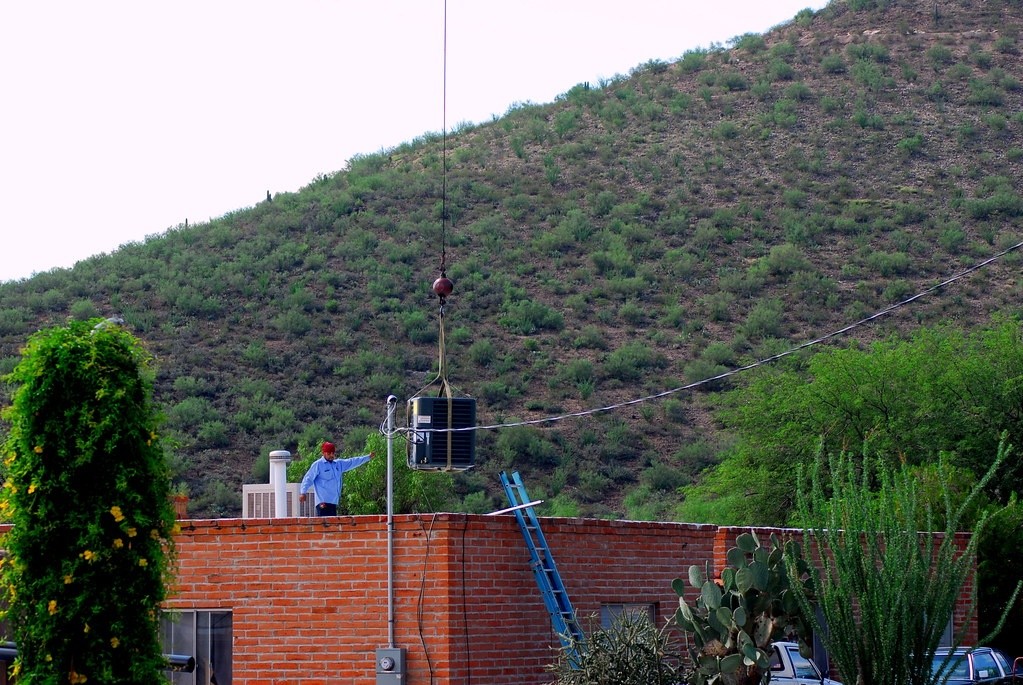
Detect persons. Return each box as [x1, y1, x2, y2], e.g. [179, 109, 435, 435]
[299, 442, 376, 517]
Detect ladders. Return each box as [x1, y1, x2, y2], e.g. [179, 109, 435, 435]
[498, 470, 588, 668]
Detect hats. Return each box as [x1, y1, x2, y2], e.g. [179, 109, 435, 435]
[322, 442, 336, 453]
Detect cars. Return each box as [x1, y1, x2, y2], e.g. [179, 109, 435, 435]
[762, 642, 843, 685]
[908, 645, 1023, 685]
[0, 640, 26, 685]
